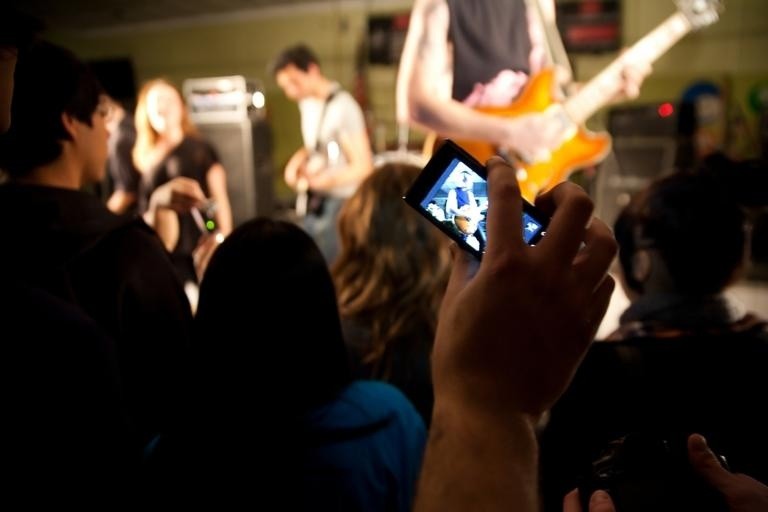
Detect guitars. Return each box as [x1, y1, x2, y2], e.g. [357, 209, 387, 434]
[293, 142, 345, 218]
[423, 0, 725, 220]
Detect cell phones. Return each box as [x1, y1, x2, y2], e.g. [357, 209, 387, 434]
[403, 138, 555, 266]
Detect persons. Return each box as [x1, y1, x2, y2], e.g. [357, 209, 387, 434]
[391, 0, 657, 206]
[444, 172, 487, 254]
[107, 79, 233, 316]
[271, 45, 375, 273]
[1, 35, 768, 511]
[425, 201, 444, 223]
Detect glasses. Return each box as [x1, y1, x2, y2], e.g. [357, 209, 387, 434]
[97, 103, 117, 124]
